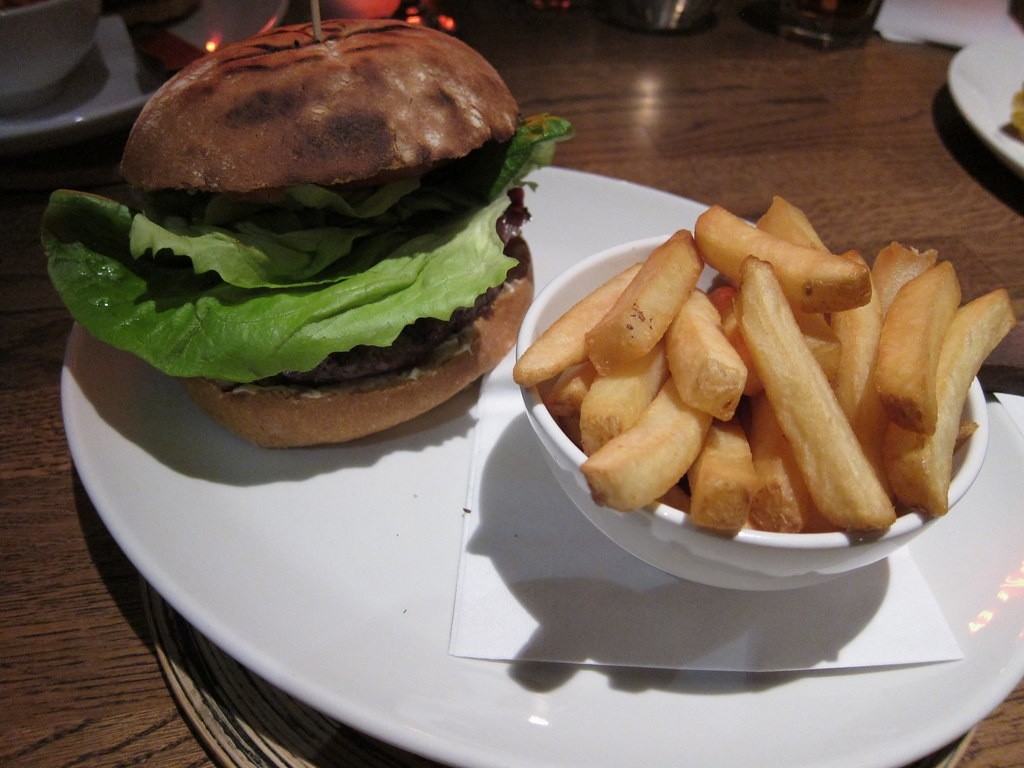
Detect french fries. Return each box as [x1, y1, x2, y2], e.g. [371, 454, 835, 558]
[515, 196, 1013, 535]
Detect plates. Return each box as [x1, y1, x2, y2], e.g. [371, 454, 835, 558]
[0, 0, 293, 147]
[947, 29, 1024, 173]
[61, 161, 1023, 768]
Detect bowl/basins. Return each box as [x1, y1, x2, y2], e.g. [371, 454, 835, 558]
[0, 0, 103, 110]
[517, 233, 992, 592]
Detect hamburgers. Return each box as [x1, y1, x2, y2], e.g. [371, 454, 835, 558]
[43, 25, 570, 449]
